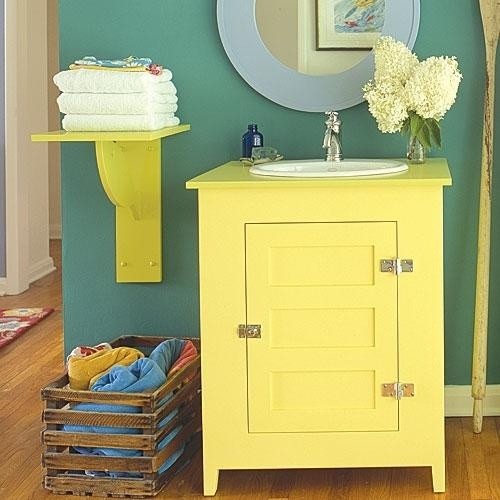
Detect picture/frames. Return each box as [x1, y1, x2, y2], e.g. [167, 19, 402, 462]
[316, 1, 384, 52]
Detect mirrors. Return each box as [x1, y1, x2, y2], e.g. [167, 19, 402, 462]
[216, 0, 422, 114]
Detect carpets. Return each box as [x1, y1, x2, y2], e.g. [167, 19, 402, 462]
[1, 307, 55, 347]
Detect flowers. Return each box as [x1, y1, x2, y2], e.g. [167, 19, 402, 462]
[361, 35, 464, 158]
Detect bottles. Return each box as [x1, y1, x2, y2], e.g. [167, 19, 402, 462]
[241, 124, 263, 160]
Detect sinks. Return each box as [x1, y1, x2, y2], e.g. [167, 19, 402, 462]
[249, 156, 409, 178]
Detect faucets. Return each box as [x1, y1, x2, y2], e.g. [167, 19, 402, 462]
[321, 111, 344, 162]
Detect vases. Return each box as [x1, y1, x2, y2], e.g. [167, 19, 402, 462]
[407, 125, 426, 164]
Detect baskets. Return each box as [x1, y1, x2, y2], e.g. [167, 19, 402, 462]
[39, 336, 202, 498]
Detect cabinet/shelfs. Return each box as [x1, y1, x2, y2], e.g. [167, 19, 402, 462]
[196, 190, 445, 496]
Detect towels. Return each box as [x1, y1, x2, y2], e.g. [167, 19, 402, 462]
[54, 55, 180, 131]
